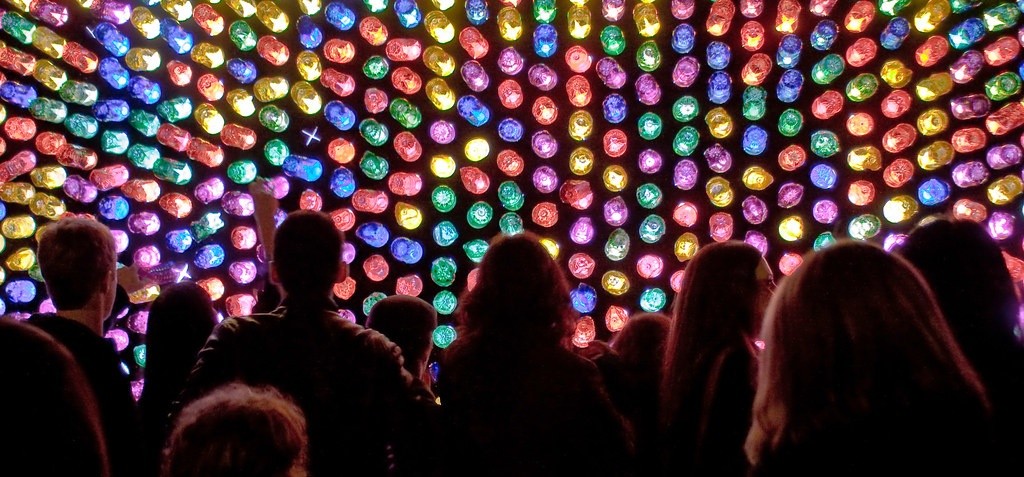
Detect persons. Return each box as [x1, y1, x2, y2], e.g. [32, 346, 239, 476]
[742, 241, 1012, 477]
[20, 217, 137, 477]
[438, 233, 637, 477]
[896, 218, 1024, 444]
[160, 382, 310, 477]
[590, 312, 670, 477]
[136, 282, 219, 477]
[166, 210, 438, 477]
[659, 241, 776, 477]
[0, 314, 111, 477]
[365, 294, 438, 394]
